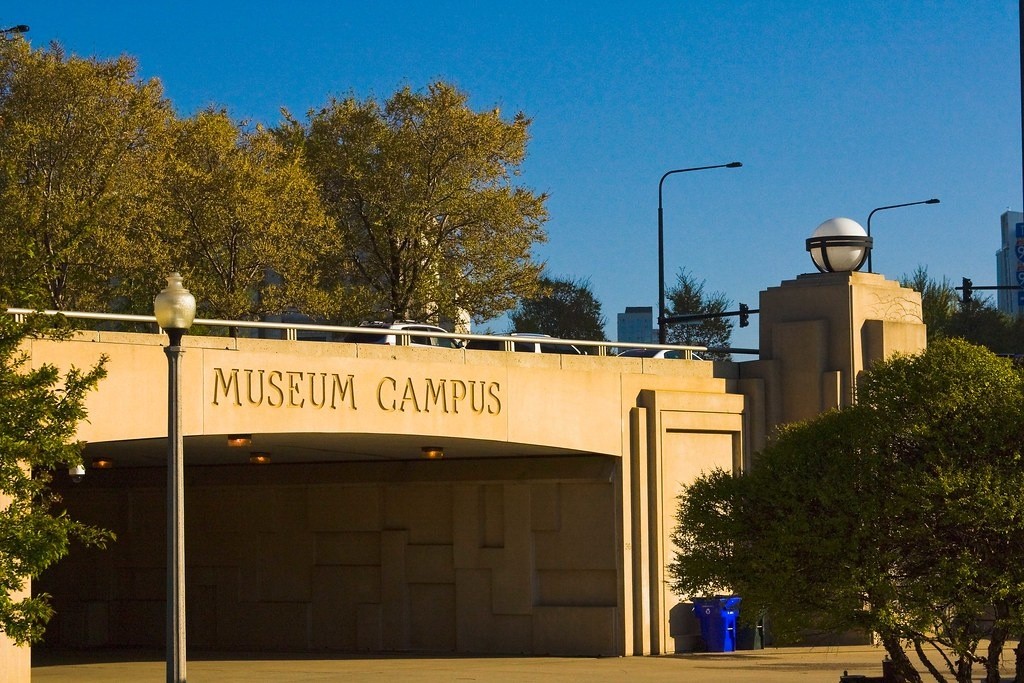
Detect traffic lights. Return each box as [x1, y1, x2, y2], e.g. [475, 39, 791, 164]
[739, 302, 749, 328]
[963, 277, 973, 304]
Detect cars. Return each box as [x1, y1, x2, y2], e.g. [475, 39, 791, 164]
[616, 349, 704, 362]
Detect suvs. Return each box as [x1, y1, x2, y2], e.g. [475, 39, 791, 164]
[466, 333, 587, 356]
[343, 319, 466, 349]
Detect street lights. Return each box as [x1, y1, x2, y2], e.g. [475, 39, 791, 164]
[155, 272, 197, 683]
[657, 162, 743, 345]
[868, 199, 940, 274]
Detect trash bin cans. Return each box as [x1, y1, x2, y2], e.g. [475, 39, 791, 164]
[689, 595, 742, 652]
